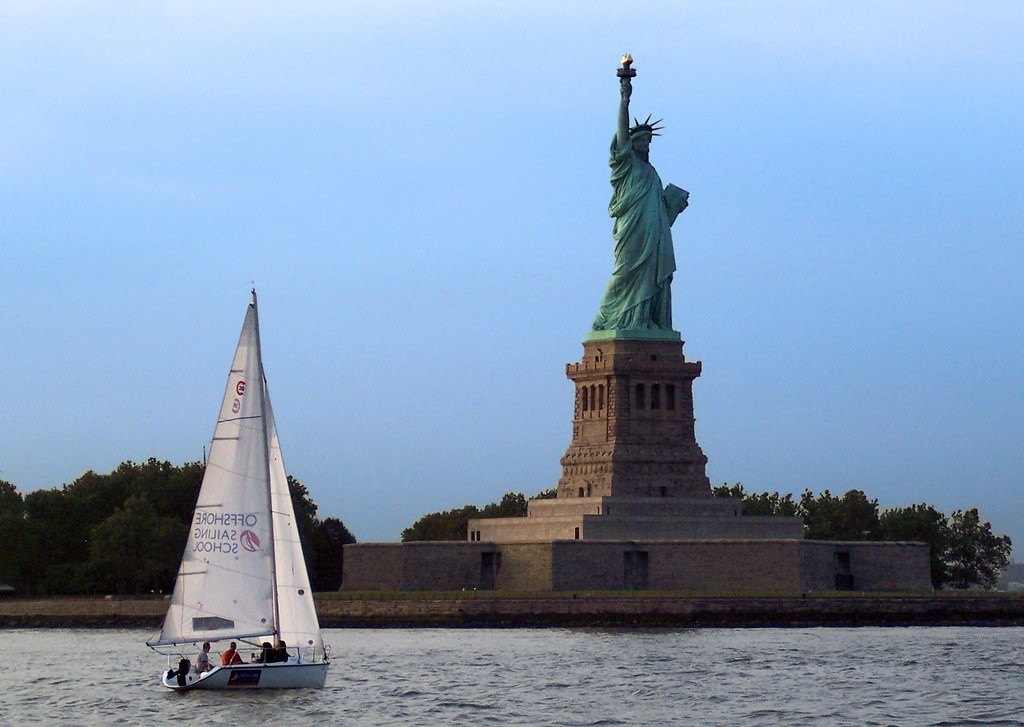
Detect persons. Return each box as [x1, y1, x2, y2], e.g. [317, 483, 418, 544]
[194, 642, 215, 673]
[592, 79, 688, 329]
[256, 642, 282, 663]
[275, 640, 291, 663]
[221, 641, 242, 665]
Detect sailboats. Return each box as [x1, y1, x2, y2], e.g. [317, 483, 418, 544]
[145, 288, 331, 692]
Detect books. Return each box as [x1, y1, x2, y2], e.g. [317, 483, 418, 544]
[663, 183, 690, 227]
